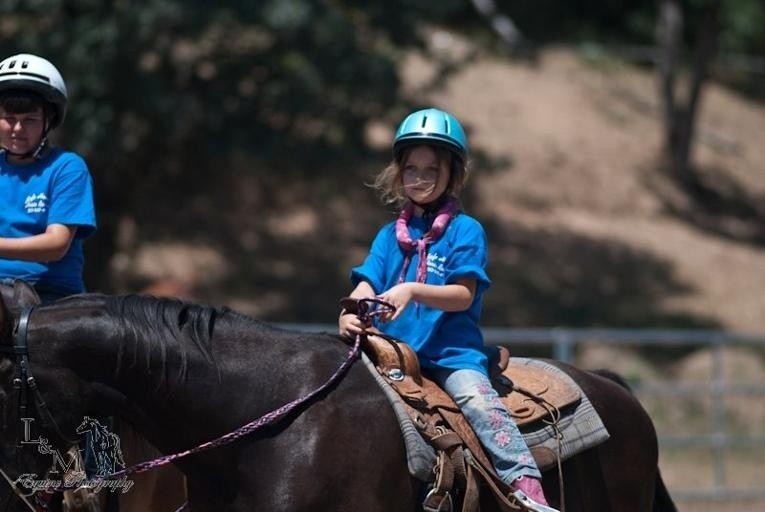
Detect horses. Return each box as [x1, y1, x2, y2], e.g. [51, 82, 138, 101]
[0, 278, 679, 512]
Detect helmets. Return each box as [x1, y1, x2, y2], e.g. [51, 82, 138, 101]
[392, 108, 468, 165]
[0, 53, 68, 129]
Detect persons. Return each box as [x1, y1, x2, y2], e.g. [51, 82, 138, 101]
[336, 105, 553, 510]
[0, 52, 100, 310]
